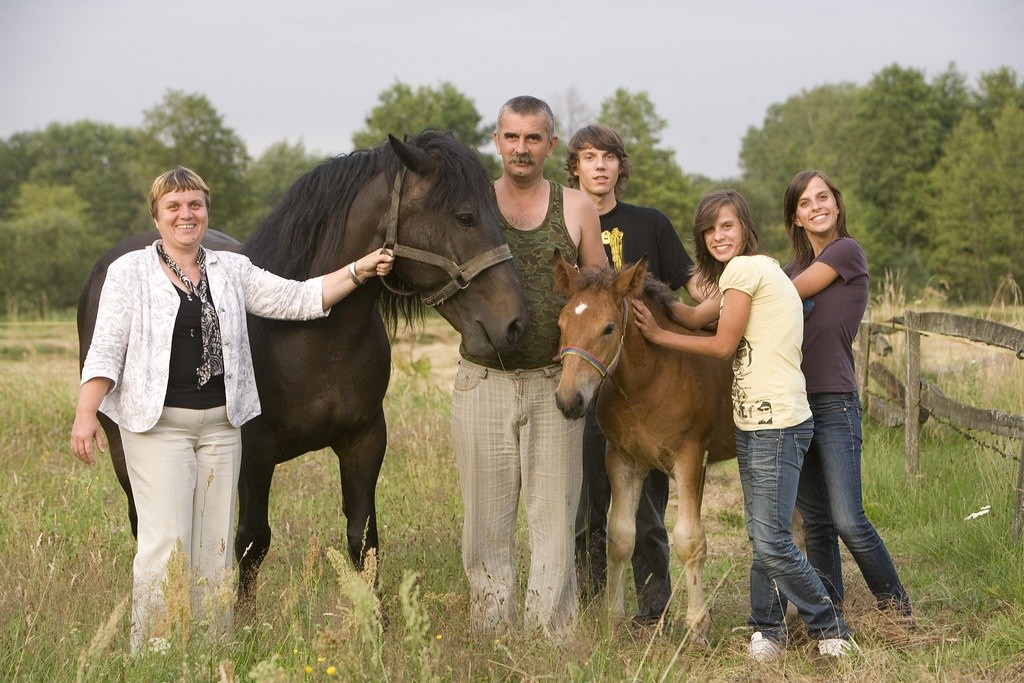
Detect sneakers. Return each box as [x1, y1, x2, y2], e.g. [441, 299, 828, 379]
[747, 631, 781, 665]
[817, 636, 851, 657]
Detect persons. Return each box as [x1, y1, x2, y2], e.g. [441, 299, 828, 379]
[70, 169, 394, 655]
[451, 94, 609, 640]
[563, 125, 716, 629]
[780, 172, 911, 618]
[631, 190, 849, 662]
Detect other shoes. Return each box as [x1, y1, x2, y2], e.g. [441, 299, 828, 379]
[630, 606, 669, 631]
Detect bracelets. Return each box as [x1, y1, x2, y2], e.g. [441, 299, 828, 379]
[349, 262, 367, 286]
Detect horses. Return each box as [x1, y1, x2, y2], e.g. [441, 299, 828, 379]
[551, 246, 740, 650]
[76, 125, 529, 635]
